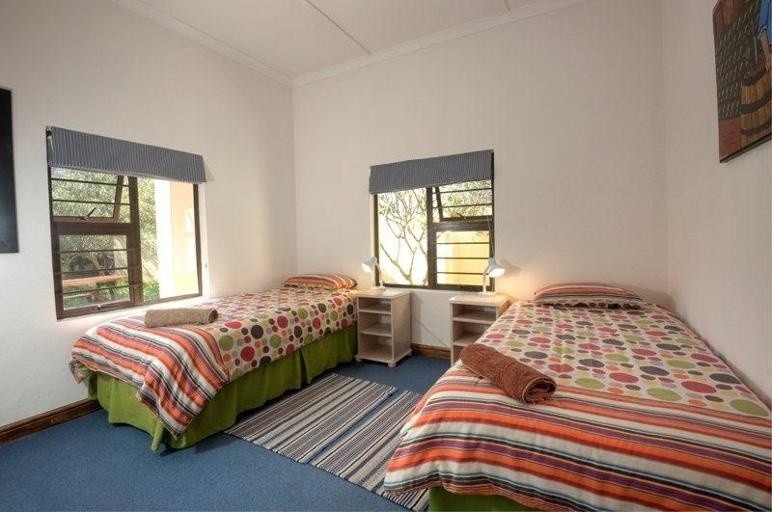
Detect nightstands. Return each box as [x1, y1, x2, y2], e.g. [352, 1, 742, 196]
[448, 296, 508, 367]
[354, 288, 412, 367]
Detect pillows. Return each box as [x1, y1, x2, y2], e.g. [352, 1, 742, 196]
[281, 270, 358, 287]
[534, 283, 651, 310]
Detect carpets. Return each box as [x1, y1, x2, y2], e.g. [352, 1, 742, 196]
[220, 370, 430, 512]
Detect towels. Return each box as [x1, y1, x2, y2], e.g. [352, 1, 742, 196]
[143, 307, 217, 326]
[458, 342, 558, 404]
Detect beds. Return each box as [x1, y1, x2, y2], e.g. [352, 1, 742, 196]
[71, 287, 360, 450]
[383, 296, 772, 510]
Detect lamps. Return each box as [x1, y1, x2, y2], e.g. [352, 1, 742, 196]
[363, 254, 388, 292]
[480, 256, 505, 297]
